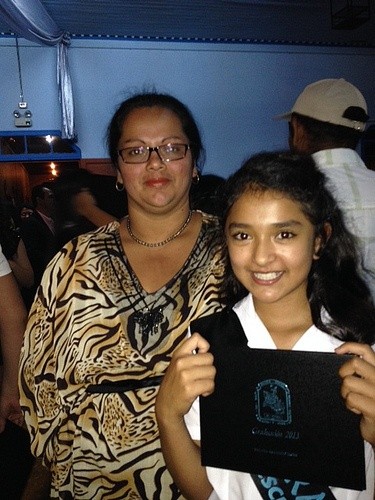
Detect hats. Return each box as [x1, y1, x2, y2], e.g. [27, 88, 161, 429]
[272, 78, 370, 133]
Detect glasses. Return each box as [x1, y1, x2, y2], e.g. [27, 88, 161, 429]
[118, 143, 193, 164]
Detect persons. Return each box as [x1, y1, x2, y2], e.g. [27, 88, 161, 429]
[154, 150, 375, 500]
[269, 79, 375, 338]
[17, 203, 34, 223]
[18, 93, 227, 500]
[0, 227, 34, 288]
[21, 184, 58, 308]
[54, 166, 119, 255]
[0, 241, 28, 431]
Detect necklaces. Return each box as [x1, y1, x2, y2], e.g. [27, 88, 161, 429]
[127, 209, 192, 247]
